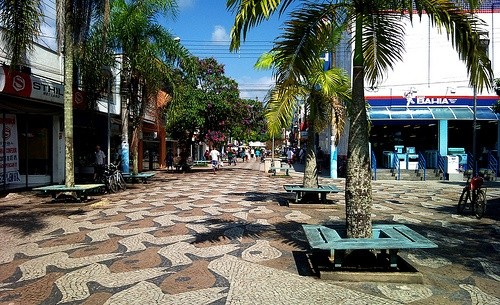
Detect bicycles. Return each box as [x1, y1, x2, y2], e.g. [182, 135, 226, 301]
[94, 159, 126, 193]
[456, 175, 487, 219]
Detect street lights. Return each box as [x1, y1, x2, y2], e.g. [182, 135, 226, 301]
[105, 37, 180, 170]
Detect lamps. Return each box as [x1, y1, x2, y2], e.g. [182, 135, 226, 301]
[447, 86, 456, 94]
[408, 86, 417, 94]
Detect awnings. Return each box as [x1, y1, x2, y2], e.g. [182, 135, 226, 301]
[367, 107, 500, 120]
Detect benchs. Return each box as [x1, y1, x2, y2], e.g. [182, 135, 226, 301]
[269, 166, 293, 175]
[300, 224, 439, 270]
[124, 170, 159, 183]
[282, 184, 341, 204]
[32, 184, 107, 200]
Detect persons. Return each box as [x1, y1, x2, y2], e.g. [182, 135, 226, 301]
[286, 146, 323, 172]
[204, 147, 220, 174]
[164, 149, 174, 171]
[227, 146, 260, 165]
[93, 146, 106, 182]
